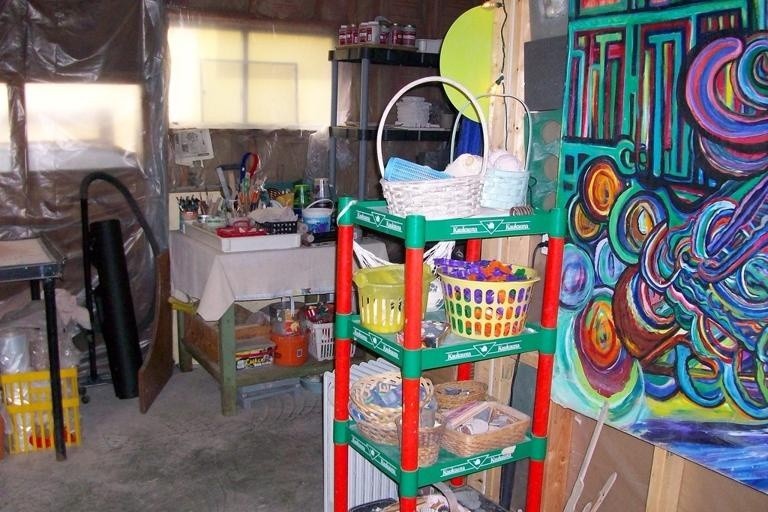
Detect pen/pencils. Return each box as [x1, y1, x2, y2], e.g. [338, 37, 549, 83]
[175, 194, 201, 213]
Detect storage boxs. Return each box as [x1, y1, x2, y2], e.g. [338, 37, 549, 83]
[182, 312, 271, 365]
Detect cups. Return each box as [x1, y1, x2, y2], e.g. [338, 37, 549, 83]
[439, 112, 454, 128]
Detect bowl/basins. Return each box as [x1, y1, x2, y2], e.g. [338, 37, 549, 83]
[300, 372, 322, 394]
[179, 211, 199, 221]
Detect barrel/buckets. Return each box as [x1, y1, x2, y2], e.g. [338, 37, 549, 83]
[271, 334, 311, 367]
[299, 198, 334, 233]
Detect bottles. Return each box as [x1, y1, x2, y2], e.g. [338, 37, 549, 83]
[336, 21, 416, 47]
[293, 177, 334, 222]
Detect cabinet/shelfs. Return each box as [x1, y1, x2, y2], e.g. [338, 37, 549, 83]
[328, 46, 462, 201]
[335, 196, 568, 512]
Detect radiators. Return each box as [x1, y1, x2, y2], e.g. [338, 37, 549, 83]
[323, 358, 401, 512]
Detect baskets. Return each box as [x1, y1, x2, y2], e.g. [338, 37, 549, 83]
[306, 320, 361, 362]
[352, 263, 436, 334]
[348, 372, 533, 469]
[0, 367, 81, 456]
[385, 157, 448, 182]
[380, 482, 472, 512]
[255, 218, 298, 235]
[375, 76, 485, 219]
[436, 263, 541, 340]
[448, 94, 533, 211]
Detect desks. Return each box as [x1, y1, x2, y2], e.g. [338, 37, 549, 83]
[0, 230, 67, 463]
[167, 229, 389, 418]
